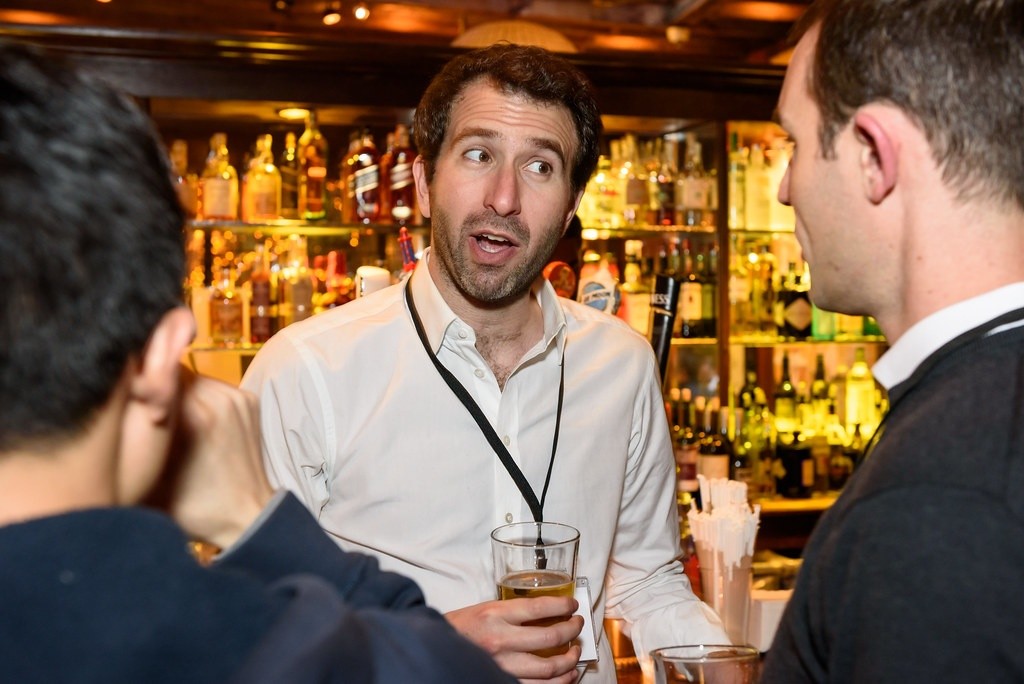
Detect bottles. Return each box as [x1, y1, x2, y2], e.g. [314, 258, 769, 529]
[543, 130, 888, 341]
[664, 345, 887, 602]
[167, 111, 423, 349]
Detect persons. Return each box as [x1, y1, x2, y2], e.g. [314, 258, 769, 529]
[0, 45, 521, 684]
[240, 42, 756, 684]
[776, 0, 1024, 684]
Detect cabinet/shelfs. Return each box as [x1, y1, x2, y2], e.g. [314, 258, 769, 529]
[143, 96, 896, 511]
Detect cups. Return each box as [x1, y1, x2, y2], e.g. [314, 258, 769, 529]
[649, 644, 760, 684]
[490, 521, 580, 657]
